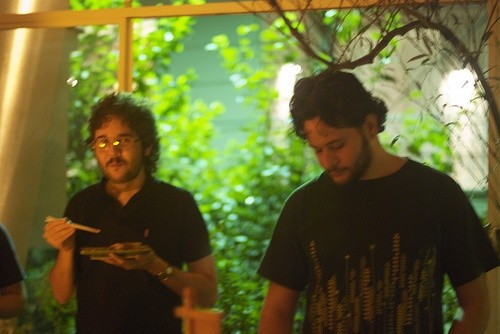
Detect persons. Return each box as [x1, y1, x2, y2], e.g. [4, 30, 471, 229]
[44, 89, 217, 334]
[0, 225, 28, 318]
[257, 71, 500, 334]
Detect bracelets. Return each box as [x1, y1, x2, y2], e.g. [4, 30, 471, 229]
[157, 266, 173, 282]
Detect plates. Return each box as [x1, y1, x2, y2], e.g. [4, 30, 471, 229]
[80, 245, 154, 256]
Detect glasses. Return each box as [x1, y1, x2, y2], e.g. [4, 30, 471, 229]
[90, 137, 142, 152]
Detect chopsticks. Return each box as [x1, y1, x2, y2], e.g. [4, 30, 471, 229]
[45, 212, 102, 234]
